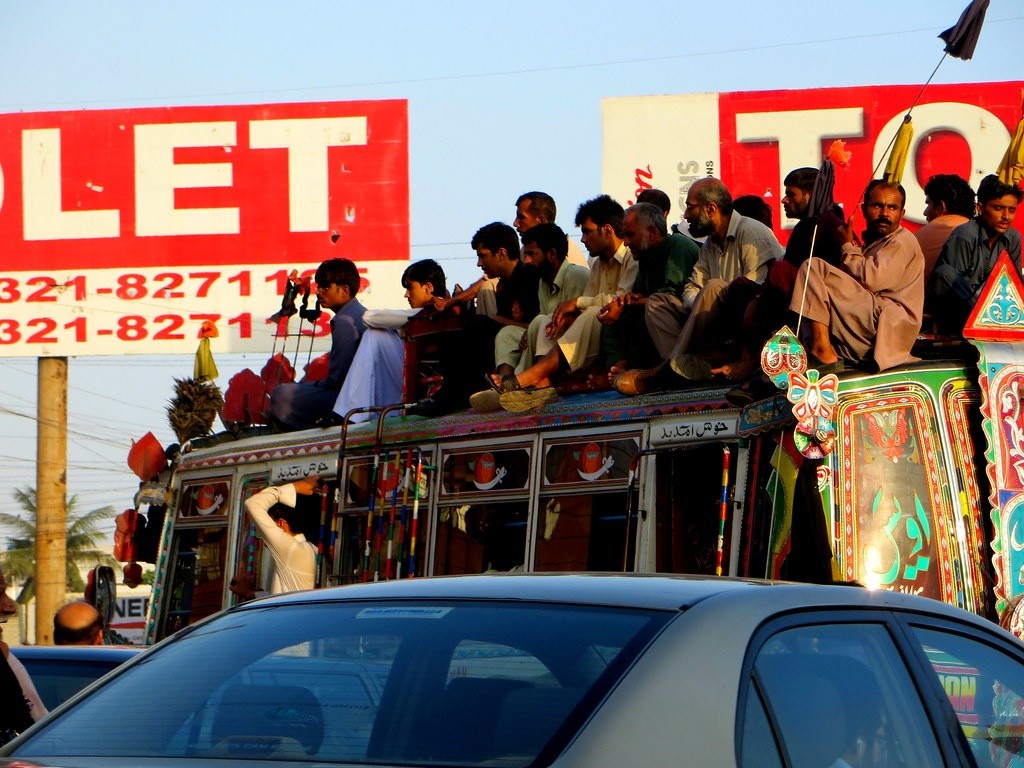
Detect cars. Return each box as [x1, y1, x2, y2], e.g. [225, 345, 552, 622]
[0, 643, 394, 767]
[0, 568, 1024, 768]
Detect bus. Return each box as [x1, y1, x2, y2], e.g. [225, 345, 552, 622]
[78, 348, 1024, 701]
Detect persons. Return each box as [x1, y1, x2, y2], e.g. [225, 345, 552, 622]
[243, 167, 1024, 656]
[53, 603, 104, 647]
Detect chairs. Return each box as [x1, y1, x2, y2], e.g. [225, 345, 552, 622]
[210, 685, 324, 755]
[405, 676, 596, 766]
[743, 653, 848, 768]
